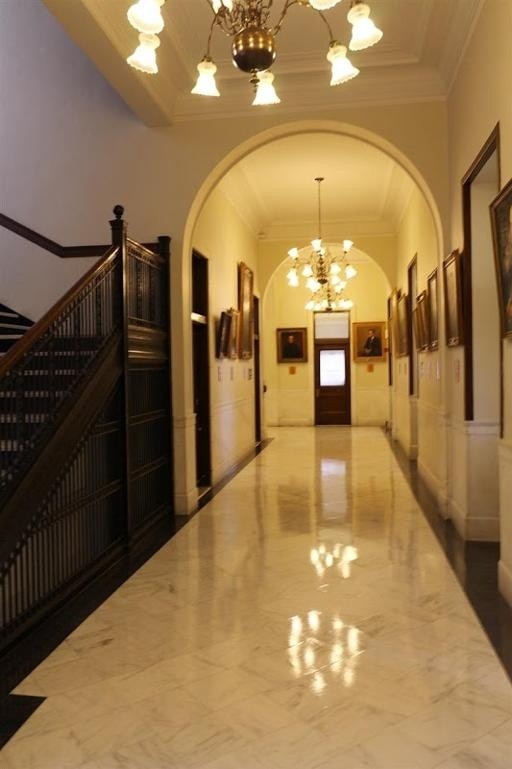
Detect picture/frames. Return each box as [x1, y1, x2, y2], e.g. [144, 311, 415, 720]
[276, 328, 308, 363]
[391, 248, 462, 357]
[488, 178, 512, 338]
[352, 322, 387, 362]
[215, 260, 254, 360]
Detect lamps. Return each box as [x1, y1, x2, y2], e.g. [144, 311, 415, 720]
[126, 0, 383, 106]
[287, 177, 356, 291]
[305, 284, 352, 311]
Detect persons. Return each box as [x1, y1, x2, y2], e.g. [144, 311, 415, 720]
[283, 335, 301, 357]
[363, 329, 380, 357]
